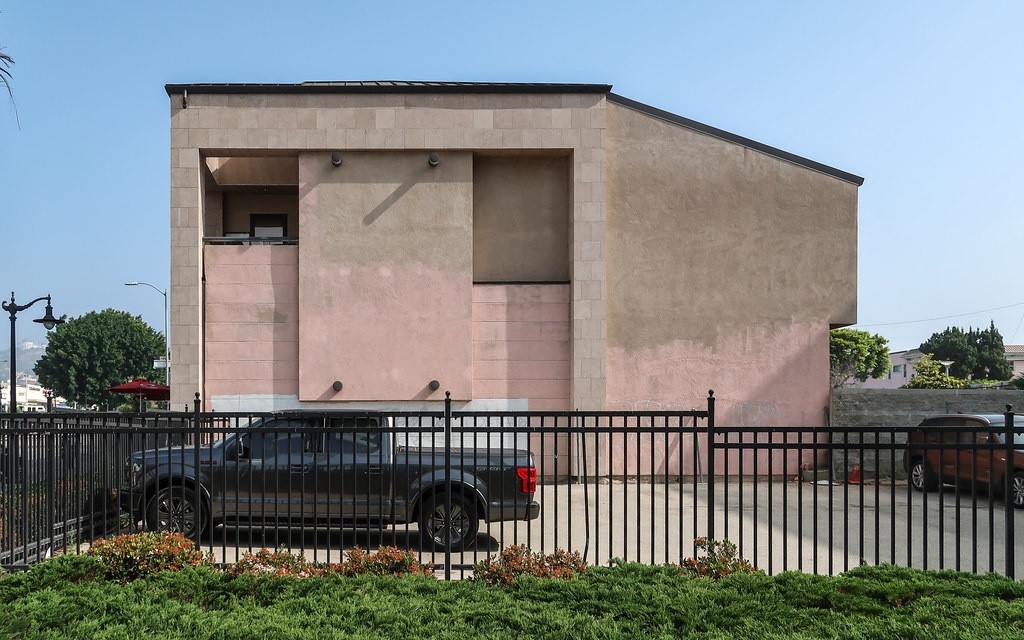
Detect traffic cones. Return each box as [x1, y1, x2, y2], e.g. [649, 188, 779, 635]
[848, 456, 860, 483]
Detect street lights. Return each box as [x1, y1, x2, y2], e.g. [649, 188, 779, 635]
[940, 358, 955, 377]
[2, 291, 66, 484]
[125, 282, 169, 411]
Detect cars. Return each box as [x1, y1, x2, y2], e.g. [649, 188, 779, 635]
[903, 413, 1024, 510]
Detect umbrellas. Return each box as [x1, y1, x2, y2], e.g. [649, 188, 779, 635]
[104, 378, 170, 412]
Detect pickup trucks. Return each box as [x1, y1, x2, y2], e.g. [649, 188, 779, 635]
[120, 409, 540, 553]
